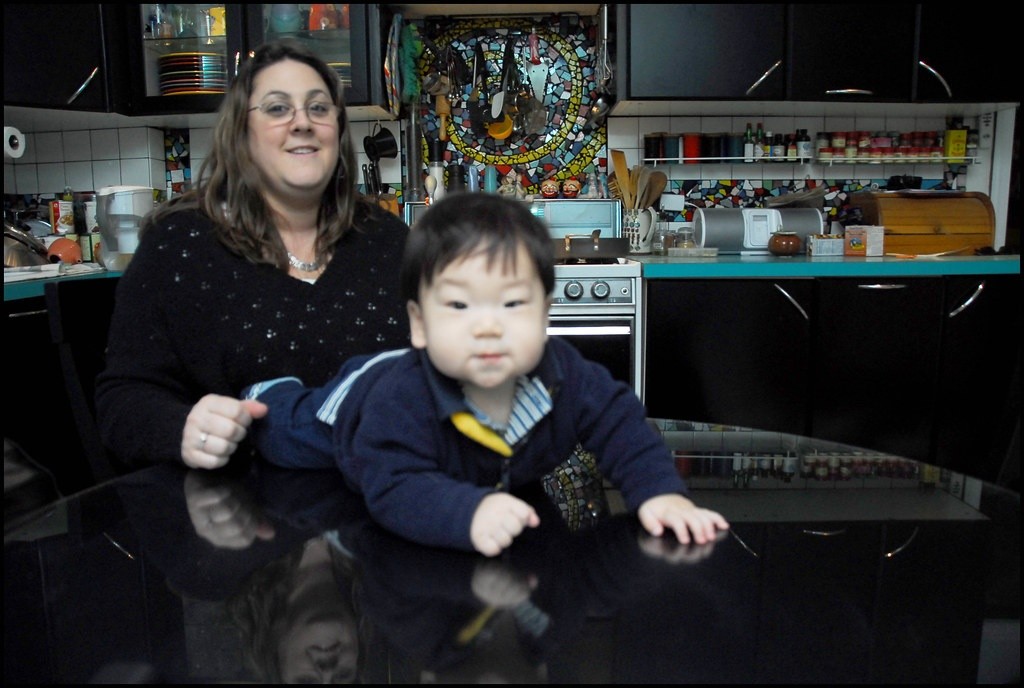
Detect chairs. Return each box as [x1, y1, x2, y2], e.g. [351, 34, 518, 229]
[43, 276, 122, 482]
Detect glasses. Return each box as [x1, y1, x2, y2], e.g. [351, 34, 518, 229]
[246, 101, 343, 126]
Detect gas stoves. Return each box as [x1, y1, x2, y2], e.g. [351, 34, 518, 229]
[404, 201, 642, 277]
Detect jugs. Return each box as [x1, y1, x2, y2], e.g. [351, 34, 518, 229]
[96, 185, 154, 271]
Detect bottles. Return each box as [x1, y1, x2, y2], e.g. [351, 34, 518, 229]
[653, 228, 695, 256]
[743, 118, 945, 165]
[731, 451, 915, 489]
[429, 140, 445, 201]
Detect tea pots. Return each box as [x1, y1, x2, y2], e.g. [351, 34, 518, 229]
[48, 236, 84, 265]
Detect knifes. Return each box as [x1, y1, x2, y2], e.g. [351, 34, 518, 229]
[362, 164, 382, 198]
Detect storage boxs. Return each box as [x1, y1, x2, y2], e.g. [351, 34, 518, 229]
[845, 225, 885, 258]
[812, 239, 844, 256]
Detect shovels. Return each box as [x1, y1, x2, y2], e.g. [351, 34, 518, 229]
[610, 149, 631, 211]
[632, 165, 667, 209]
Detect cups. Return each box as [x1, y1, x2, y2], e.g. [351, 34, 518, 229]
[623, 207, 657, 254]
[363, 122, 398, 163]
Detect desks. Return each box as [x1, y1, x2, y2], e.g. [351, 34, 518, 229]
[3, 410, 1020, 684]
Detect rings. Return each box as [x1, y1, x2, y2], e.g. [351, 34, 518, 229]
[196, 432, 207, 450]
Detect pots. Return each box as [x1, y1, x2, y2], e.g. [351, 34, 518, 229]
[549, 228, 629, 261]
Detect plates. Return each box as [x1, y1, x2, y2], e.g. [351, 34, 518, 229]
[324, 62, 352, 89]
[157, 52, 226, 95]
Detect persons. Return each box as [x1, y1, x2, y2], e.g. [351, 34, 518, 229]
[95, 40, 590, 471]
[237, 193, 729, 558]
[109, 462, 729, 688]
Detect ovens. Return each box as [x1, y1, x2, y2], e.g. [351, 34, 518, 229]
[545, 277, 643, 400]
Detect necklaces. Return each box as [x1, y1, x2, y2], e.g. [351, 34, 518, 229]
[220, 200, 331, 272]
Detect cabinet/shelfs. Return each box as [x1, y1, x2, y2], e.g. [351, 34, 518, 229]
[112, 0, 394, 122]
[628, 3, 1021, 492]
[1, 2, 112, 112]
[3, 295, 90, 495]
